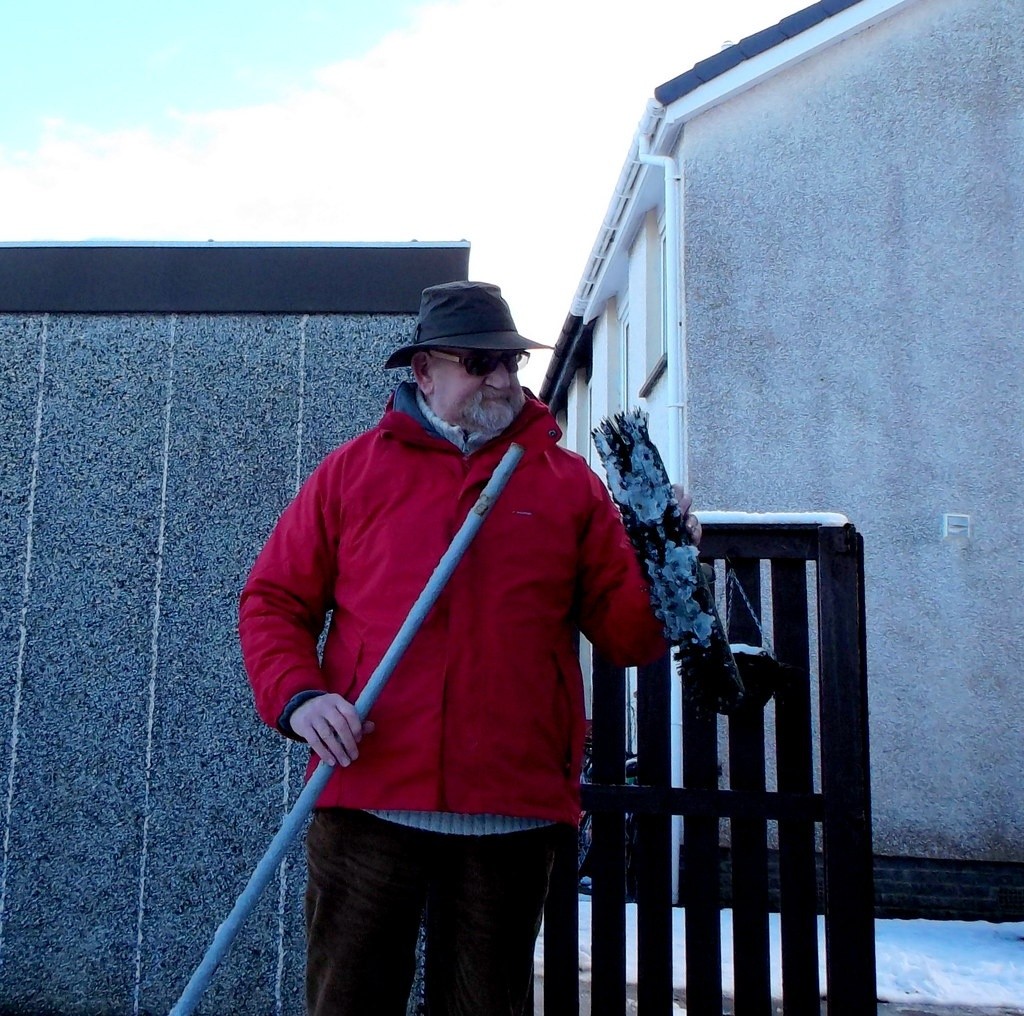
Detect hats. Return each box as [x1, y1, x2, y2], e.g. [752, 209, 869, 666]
[385, 280, 555, 369]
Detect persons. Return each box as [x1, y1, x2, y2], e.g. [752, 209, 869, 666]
[237, 282, 704, 1016]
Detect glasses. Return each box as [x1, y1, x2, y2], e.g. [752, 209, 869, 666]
[425, 349, 530, 377]
